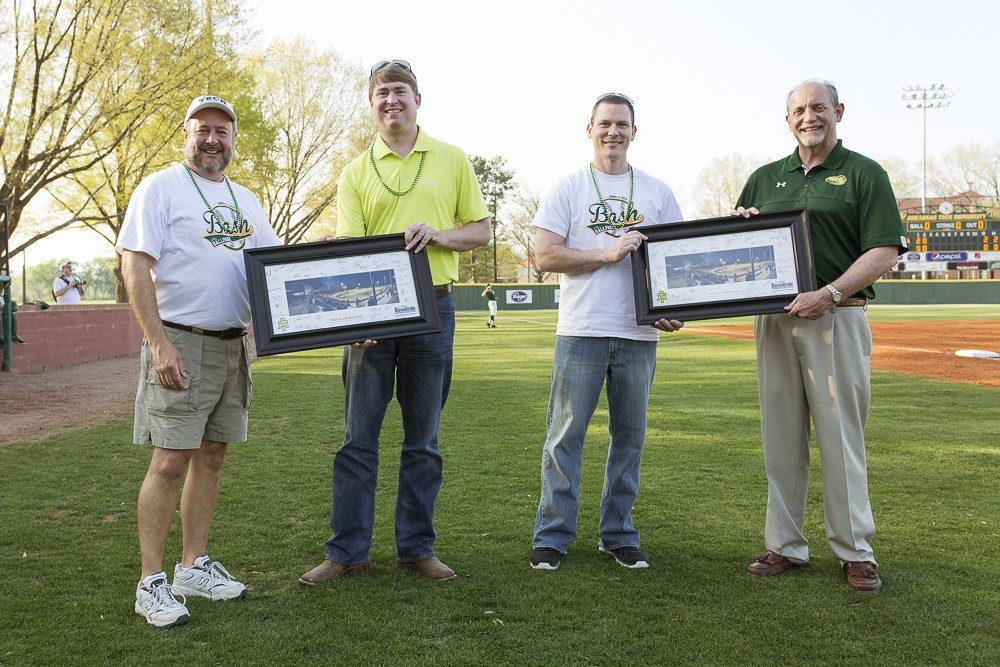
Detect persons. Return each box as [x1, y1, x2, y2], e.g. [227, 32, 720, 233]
[298, 59, 491, 583]
[482, 283, 498, 328]
[529, 94, 686, 569]
[729, 79, 909, 587]
[115, 96, 286, 628]
[0, 297, 26, 345]
[53, 261, 85, 304]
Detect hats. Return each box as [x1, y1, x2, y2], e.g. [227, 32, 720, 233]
[184, 96, 237, 126]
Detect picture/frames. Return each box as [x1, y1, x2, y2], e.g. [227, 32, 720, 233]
[242, 233, 441, 358]
[631, 210, 817, 326]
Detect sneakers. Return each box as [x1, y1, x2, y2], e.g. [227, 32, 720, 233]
[598, 544, 650, 568]
[529, 546, 560, 570]
[298, 559, 370, 587]
[135, 571, 190, 628]
[397, 556, 457, 582]
[748, 551, 795, 578]
[843, 562, 882, 592]
[171, 554, 247, 601]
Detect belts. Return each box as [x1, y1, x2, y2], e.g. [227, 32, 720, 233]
[434, 284, 452, 297]
[161, 319, 246, 340]
[836, 298, 866, 307]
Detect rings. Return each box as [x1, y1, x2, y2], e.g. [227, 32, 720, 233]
[418, 233, 424, 237]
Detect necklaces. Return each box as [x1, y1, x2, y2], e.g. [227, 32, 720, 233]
[370, 143, 427, 195]
[589, 162, 634, 232]
[182, 161, 242, 232]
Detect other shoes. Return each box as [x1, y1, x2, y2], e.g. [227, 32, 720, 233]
[492, 325, 497, 328]
[486, 322, 490, 328]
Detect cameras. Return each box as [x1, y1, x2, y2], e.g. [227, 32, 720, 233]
[74, 280, 86, 287]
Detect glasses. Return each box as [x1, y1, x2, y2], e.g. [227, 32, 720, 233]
[371, 60, 411, 77]
[597, 93, 633, 106]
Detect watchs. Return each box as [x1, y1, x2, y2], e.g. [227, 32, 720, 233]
[825, 283, 842, 304]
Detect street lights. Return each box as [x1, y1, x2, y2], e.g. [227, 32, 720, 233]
[901, 82, 955, 279]
